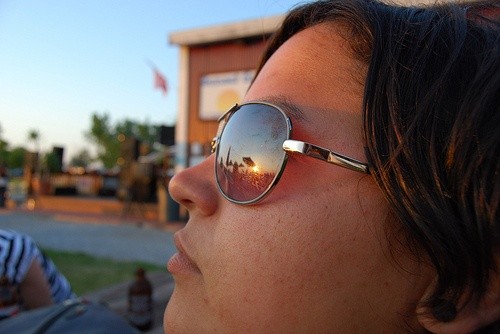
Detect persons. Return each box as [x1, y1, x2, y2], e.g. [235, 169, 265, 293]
[163, 0, 500, 334]
[0, 228, 74, 315]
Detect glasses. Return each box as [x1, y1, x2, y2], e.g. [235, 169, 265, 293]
[209, 102, 371, 205]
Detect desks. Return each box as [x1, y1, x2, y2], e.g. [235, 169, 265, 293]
[78, 270, 175, 334]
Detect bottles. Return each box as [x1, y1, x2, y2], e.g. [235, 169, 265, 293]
[126, 268, 154, 331]
